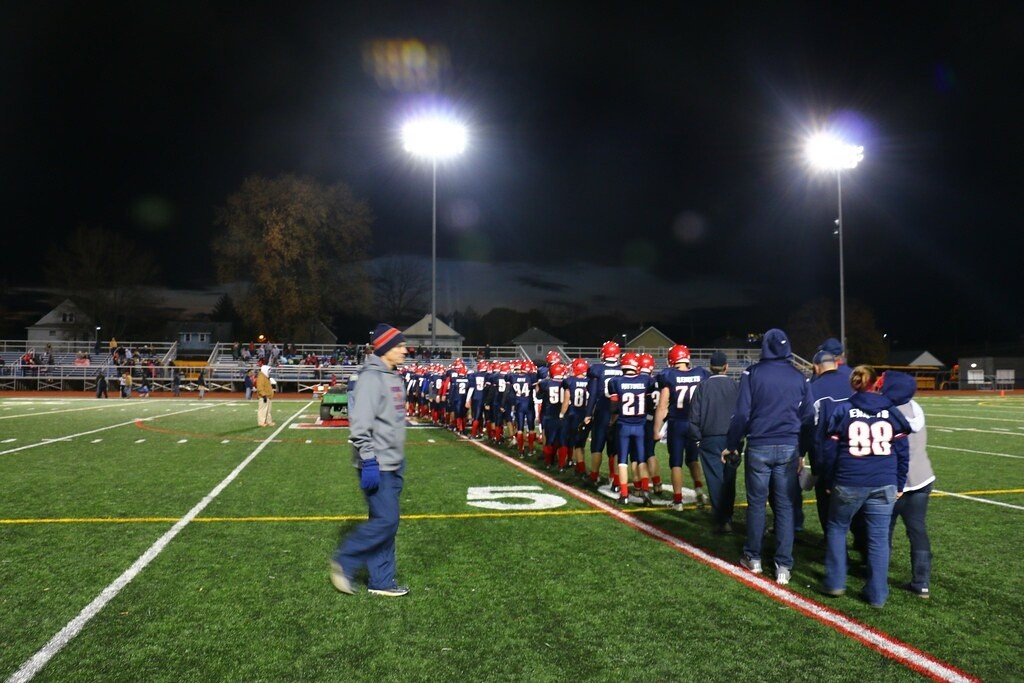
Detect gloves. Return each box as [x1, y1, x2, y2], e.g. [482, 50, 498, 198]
[360, 458, 382, 491]
[263, 396, 267, 403]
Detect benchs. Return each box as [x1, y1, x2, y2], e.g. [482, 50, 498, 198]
[564, 358, 754, 373]
[0, 352, 166, 376]
[398, 356, 523, 368]
[214, 353, 364, 380]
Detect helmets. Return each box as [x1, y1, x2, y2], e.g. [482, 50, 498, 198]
[399, 341, 692, 376]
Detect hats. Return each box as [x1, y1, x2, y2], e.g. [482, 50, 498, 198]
[822, 338, 844, 356]
[371, 324, 406, 357]
[814, 351, 835, 363]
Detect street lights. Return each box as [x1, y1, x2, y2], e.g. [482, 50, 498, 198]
[803, 124, 864, 364]
[400, 112, 469, 355]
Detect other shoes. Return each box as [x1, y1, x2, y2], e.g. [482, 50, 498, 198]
[653, 485, 663, 493]
[369, 586, 412, 597]
[820, 588, 846, 596]
[696, 495, 708, 505]
[667, 502, 684, 511]
[408, 412, 619, 493]
[773, 565, 792, 584]
[740, 556, 763, 574]
[261, 422, 276, 428]
[898, 581, 930, 598]
[616, 496, 630, 505]
[328, 564, 353, 594]
[644, 496, 653, 507]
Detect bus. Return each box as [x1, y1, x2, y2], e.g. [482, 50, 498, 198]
[873, 364, 950, 391]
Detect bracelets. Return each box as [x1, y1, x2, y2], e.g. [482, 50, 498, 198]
[559, 412, 564, 418]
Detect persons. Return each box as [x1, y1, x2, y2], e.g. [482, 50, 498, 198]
[802, 339, 935, 608]
[329, 324, 410, 596]
[721, 328, 815, 584]
[0, 337, 372, 426]
[690, 352, 745, 536]
[401, 340, 705, 512]
